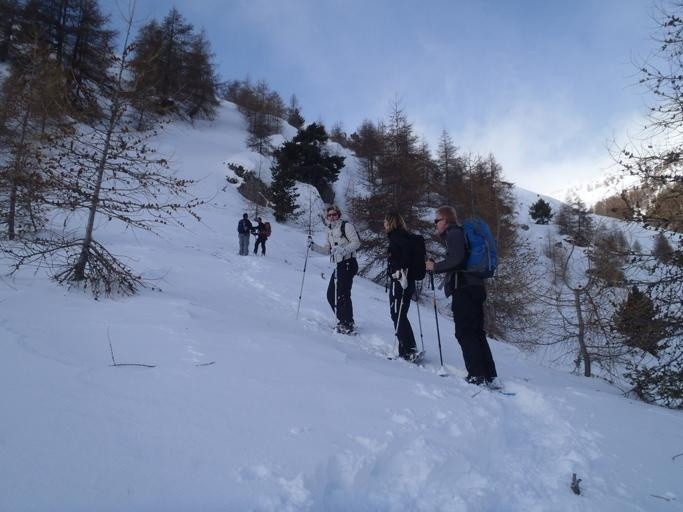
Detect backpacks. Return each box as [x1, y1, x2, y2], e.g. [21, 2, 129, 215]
[264, 222, 271, 239]
[461, 218, 498, 277]
[406, 234, 427, 281]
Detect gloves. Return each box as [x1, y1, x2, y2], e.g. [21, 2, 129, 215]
[305, 238, 314, 248]
[331, 246, 344, 256]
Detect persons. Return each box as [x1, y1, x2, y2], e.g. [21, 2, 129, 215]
[251, 216, 267, 256]
[382, 209, 426, 362]
[236, 213, 255, 256]
[425, 205, 504, 389]
[305, 205, 361, 337]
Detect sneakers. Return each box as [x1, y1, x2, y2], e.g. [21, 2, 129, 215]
[465, 375, 502, 389]
[405, 348, 425, 363]
[333, 325, 359, 335]
